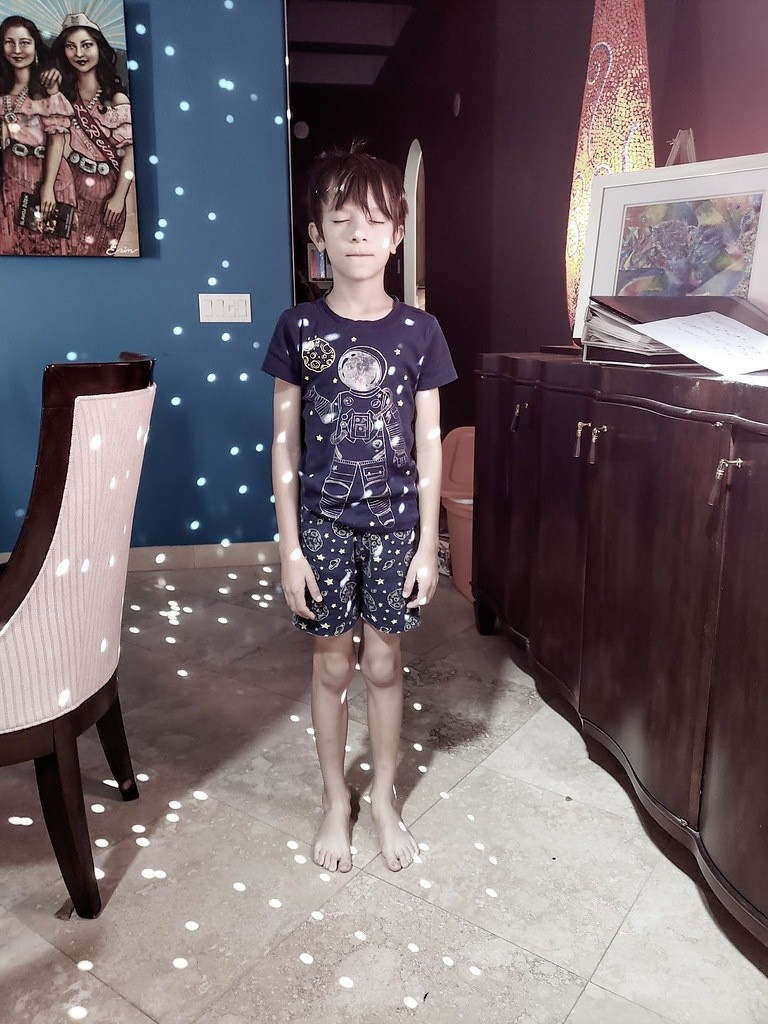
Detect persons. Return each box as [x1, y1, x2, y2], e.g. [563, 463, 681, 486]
[268, 153, 442, 873]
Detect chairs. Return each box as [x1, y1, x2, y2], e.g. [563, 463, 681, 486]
[1, 350, 158, 919]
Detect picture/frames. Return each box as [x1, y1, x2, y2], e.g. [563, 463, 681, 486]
[573, 153, 767, 344]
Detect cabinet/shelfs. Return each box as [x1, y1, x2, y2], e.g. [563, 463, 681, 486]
[477, 374, 768, 954]
